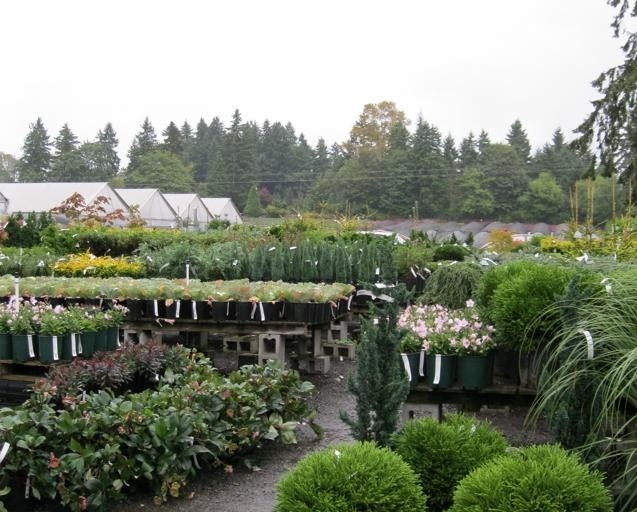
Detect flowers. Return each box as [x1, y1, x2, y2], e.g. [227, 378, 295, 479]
[396, 299, 495, 357]
[0, 298, 129, 335]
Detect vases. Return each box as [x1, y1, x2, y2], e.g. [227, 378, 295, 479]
[403, 345, 497, 390]
[0, 327, 118, 364]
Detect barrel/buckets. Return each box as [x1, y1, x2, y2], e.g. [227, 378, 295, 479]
[402, 350, 492, 393]
[0, 327, 120, 363]
